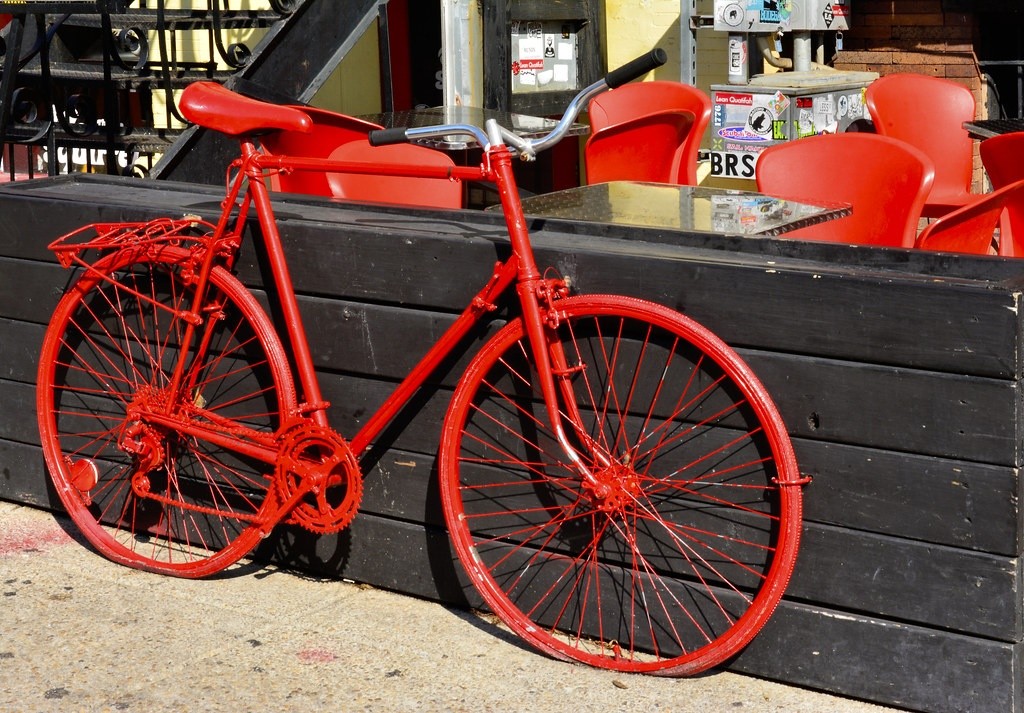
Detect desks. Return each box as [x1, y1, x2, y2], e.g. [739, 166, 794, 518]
[480, 181, 854, 236]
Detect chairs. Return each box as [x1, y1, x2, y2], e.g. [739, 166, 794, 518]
[978, 132, 1024, 189]
[755, 132, 935, 248]
[261, 102, 385, 198]
[323, 140, 461, 208]
[587, 80, 713, 185]
[585, 110, 695, 185]
[912, 176, 1024, 257]
[864, 73, 1001, 256]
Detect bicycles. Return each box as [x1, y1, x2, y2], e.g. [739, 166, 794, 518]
[33, 45, 804, 681]
